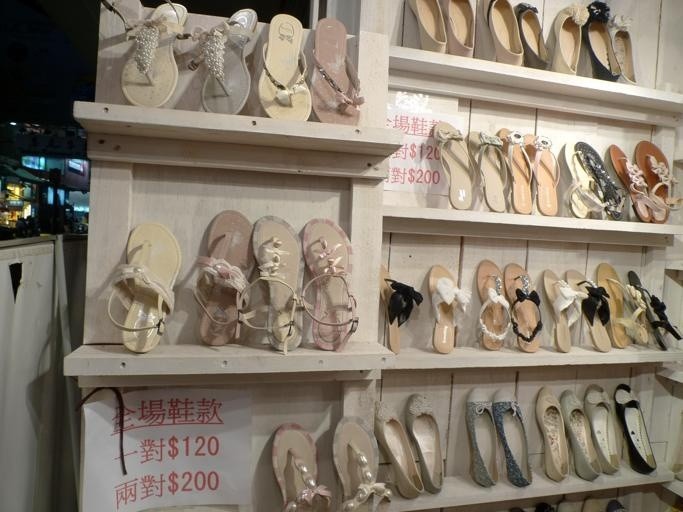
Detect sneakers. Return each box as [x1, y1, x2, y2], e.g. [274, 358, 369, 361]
[466, 385, 533, 486]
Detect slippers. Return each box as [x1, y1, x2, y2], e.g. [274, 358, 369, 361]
[311, 18, 365, 125]
[259, 12, 311, 121]
[427, 265, 471, 354]
[378, 265, 423, 354]
[433, 120, 560, 216]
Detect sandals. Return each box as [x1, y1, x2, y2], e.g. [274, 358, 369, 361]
[108, 222, 181, 354]
[564, 140, 682, 225]
[542, 263, 682, 352]
[193, 209, 358, 354]
[476, 260, 542, 353]
[189, 8, 257, 114]
[121, 1, 188, 109]
[271, 422, 331, 512]
[331, 416, 392, 512]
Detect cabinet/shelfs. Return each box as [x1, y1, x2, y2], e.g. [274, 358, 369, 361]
[62, 0, 683, 512]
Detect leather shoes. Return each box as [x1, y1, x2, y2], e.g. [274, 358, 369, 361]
[410, 0, 638, 86]
[374, 400, 425, 499]
[405, 393, 444, 494]
[615, 384, 657, 473]
[536, 385, 619, 482]
